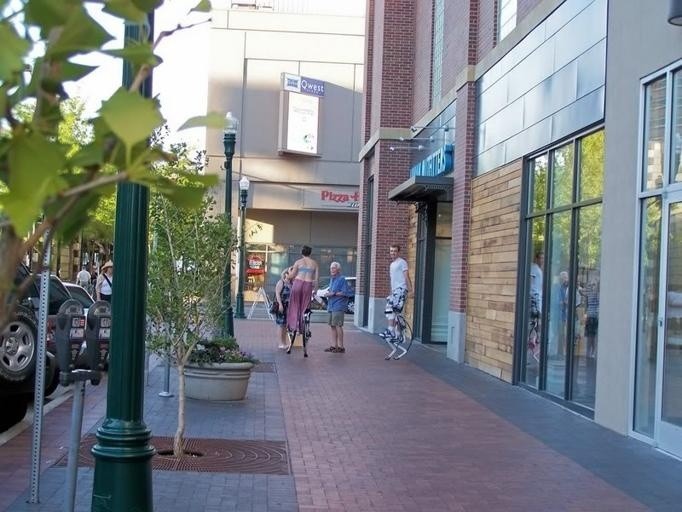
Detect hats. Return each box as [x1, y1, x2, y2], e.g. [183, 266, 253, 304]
[101, 261, 114, 269]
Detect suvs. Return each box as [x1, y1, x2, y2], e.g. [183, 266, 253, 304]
[0, 262, 114, 435]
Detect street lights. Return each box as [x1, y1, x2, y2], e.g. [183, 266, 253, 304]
[234, 174, 250, 319]
[220, 112, 236, 349]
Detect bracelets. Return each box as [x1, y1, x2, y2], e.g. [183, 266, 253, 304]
[333, 291, 337, 298]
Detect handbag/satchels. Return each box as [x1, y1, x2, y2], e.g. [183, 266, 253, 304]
[268, 301, 277, 314]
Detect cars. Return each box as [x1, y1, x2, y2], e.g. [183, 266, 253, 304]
[313, 276, 357, 315]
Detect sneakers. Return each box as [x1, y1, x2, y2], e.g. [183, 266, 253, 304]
[324, 346, 346, 353]
[379, 329, 397, 339]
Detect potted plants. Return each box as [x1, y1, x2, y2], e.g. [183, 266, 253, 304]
[185, 336, 262, 400]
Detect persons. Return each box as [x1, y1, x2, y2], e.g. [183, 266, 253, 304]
[323, 261, 352, 353]
[274, 267, 293, 350]
[528, 249, 545, 342]
[77, 259, 113, 302]
[379, 245, 414, 342]
[555, 270, 599, 360]
[284, 244, 319, 331]
